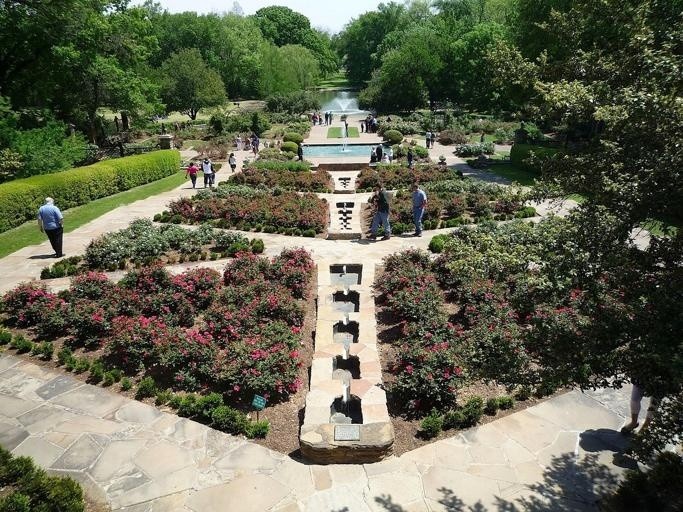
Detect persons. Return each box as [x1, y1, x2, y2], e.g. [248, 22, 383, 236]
[311, 111, 333, 126]
[236, 134, 280, 155]
[368, 141, 413, 167]
[622, 382, 661, 445]
[294, 143, 303, 161]
[37, 196, 63, 257]
[200, 156, 214, 189]
[228, 153, 235, 173]
[360, 116, 377, 133]
[365, 183, 390, 240]
[411, 182, 427, 237]
[173, 120, 193, 132]
[160, 123, 166, 135]
[184, 162, 199, 189]
[207, 159, 215, 184]
[425, 129, 430, 149]
[431, 130, 435, 149]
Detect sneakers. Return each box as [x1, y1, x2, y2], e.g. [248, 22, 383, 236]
[412, 233, 422, 237]
[56, 254, 65, 258]
[366, 234, 390, 240]
